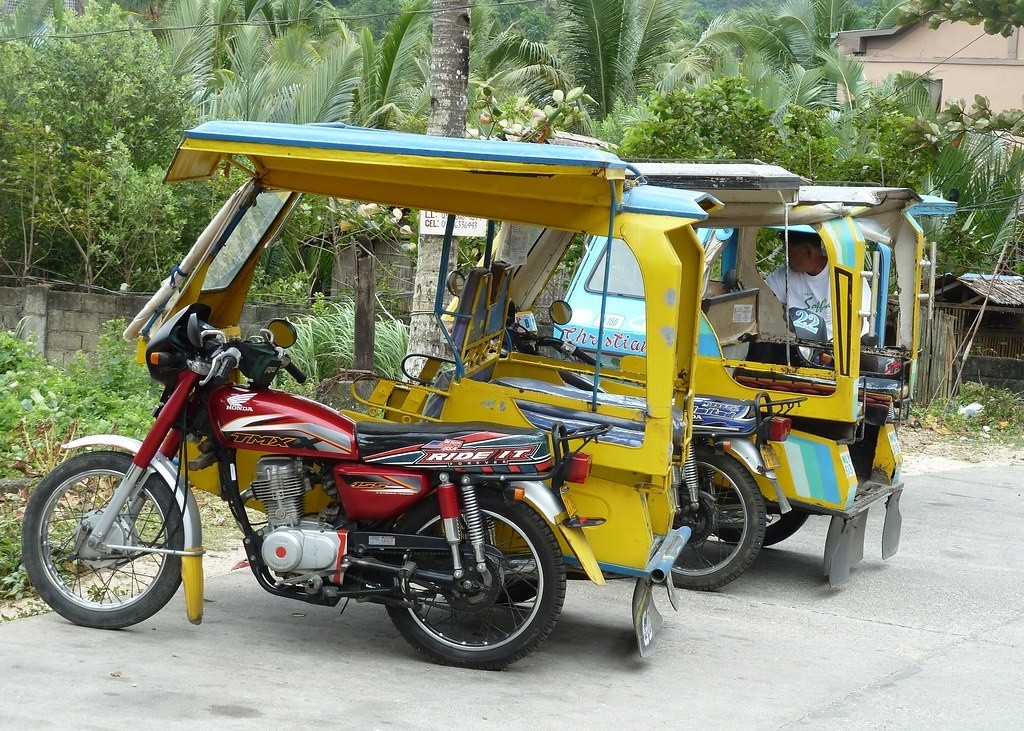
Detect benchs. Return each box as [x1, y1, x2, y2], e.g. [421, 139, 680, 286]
[703, 287, 924, 422]
[420, 259, 694, 477]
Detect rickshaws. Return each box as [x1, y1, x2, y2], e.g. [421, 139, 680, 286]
[22, 114, 722, 669]
[426, 156, 961, 589]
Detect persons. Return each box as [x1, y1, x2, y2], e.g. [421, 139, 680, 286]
[768, 231, 872, 367]
